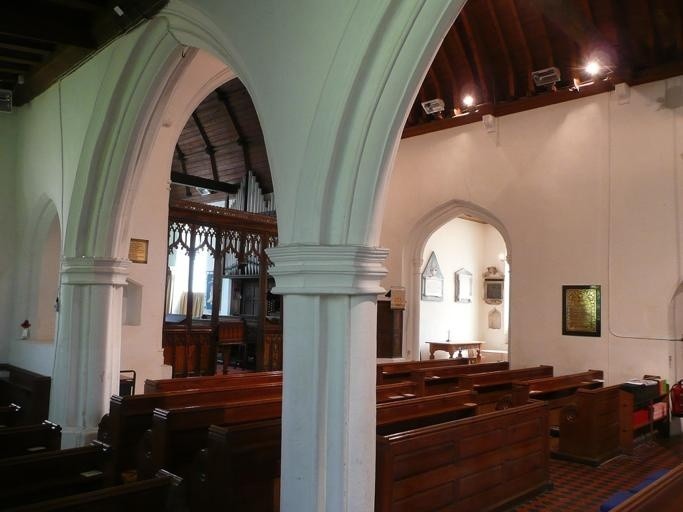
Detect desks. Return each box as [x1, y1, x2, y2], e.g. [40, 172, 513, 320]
[425, 340, 486, 366]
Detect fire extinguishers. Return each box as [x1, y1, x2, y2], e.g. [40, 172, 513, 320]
[672, 378, 683, 417]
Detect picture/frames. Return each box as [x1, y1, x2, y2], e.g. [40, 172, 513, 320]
[562, 284, 602, 337]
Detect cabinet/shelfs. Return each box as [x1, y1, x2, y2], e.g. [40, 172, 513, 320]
[378, 300, 403, 357]
